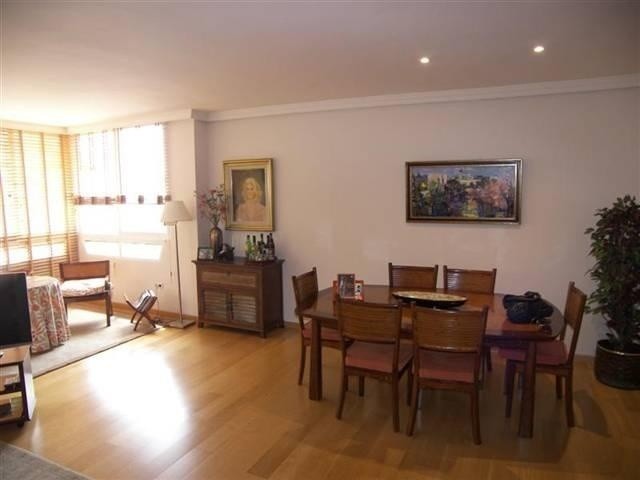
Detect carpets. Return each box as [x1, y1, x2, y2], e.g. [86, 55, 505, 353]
[30, 306, 162, 379]
[0, 438, 96, 480]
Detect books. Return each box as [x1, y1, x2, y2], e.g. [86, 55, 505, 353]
[137, 289, 155, 312]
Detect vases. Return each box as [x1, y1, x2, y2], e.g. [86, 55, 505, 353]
[208, 224, 224, 261]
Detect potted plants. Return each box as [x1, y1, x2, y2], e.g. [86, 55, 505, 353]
[580, 190, 640, 392]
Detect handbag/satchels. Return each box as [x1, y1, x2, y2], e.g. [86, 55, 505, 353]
[503, 291, 553, 324]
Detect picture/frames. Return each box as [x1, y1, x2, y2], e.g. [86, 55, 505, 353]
[221, 158, 276, 234]
[403, 157, 524, 226]
[198, 247, 215, 261]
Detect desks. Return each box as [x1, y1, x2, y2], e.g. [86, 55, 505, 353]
[26, 274, 74, 353]
[293, 282, 566, 439]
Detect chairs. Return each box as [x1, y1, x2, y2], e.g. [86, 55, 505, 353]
[59, 258, 114, 327]
[442, 264, 498, 373]
[330, 292, 414, 435]
[290, 266, 353, 394]
[407, 302, 491, 445]
[385, 262, 438, 293]
[497, 280, 588, 429]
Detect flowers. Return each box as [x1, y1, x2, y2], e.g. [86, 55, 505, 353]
[191, 182, 230, 228]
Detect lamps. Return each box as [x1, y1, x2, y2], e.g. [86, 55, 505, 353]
[158, 199, 197, 330]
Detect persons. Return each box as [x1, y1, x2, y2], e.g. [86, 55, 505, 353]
[235, 177, 265, 222]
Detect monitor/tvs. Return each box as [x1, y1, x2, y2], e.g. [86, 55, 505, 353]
[0, 272, 32, 358]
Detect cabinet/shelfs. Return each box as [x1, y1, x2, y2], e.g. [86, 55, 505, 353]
[0, 344, 38, 430]
[192, 258, 286, 338]
